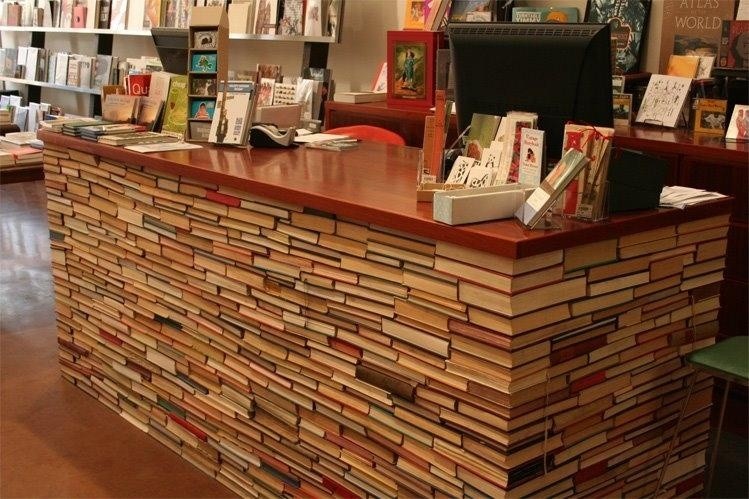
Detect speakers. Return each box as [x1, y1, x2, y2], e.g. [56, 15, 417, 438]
[609, 148, 667, 213]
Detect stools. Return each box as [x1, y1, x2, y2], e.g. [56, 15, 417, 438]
[654, 333, 749, 499]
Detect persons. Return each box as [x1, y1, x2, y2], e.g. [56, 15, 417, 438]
[401, 49, 414, 89]
[736, 109, 749, 140]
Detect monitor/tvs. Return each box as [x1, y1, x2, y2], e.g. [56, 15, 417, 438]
[447, 20, 614, 161]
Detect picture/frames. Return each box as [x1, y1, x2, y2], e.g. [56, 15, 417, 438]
[386, 30, 435, 112]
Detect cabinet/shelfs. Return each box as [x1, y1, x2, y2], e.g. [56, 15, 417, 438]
[325, 94, 749, 402]
[0, 0, 345, 121]
[183, 6, 229, 144]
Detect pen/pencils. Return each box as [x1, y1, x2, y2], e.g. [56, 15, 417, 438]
[333, 139, 364, 143]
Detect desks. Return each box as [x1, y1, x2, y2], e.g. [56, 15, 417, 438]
[36, 123, 734, 498]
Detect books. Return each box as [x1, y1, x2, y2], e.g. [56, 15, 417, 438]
[0, 0, 342, 42]
[403, 0, 451, 32]
[207, 63, 333, 147]
[333, 90, 386, 104]
[0, 45, 163, 92]
[0, 94, 62, 150]
[494, 110, 615, 230]
[451, 0, 497, 22]
[635, 73, 692, 128]
[40, 72, 188, 148]
